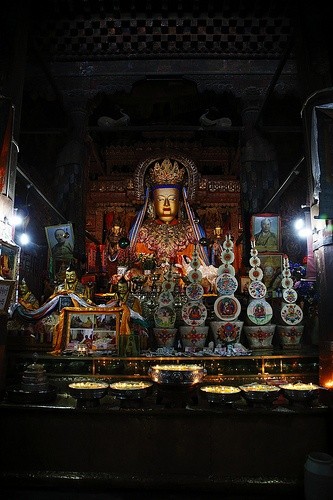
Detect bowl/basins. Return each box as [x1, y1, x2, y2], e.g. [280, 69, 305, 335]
[275, 326, 304, 346]
[109, 381, 154, 403]
[199, 384, 241, 406]
[68, 381, 109, 404]
[209, 322, 244, 346]
[278, 384, 322, 405]
[243, 325, 276, 349]
[319, 385, 333, 403]
[241, 384, 281, 403]
[178, 326, 209, 351]
[152, 328, 178, 349]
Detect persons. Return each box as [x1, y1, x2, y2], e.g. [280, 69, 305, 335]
[52, 229, 74, 256]
[162, 312, 168, 323]
[189, 309, 201, 319]
[96, 315, 116, 328]
[264, 256, 274, 267]
[255, 306, 264, 316]
[18, 278, 39, 310]
[222, 301, 233, 315]
[70, 333, 92, 345]
[93, 335, 100, 341]
[105, 333, 112, 339]
[255, 219, 278, 246]
[106, 277, 142, 315]
[70, 316, 92, 329]
[108, 156, 210, 267]
[54, 264, 86, 295]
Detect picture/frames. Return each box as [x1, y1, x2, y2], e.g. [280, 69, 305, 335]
[63, 307, 123, 354]
[45, 223, 75, 257]
[259, 253, 284, 290]
[250, 213, 283, 251]
